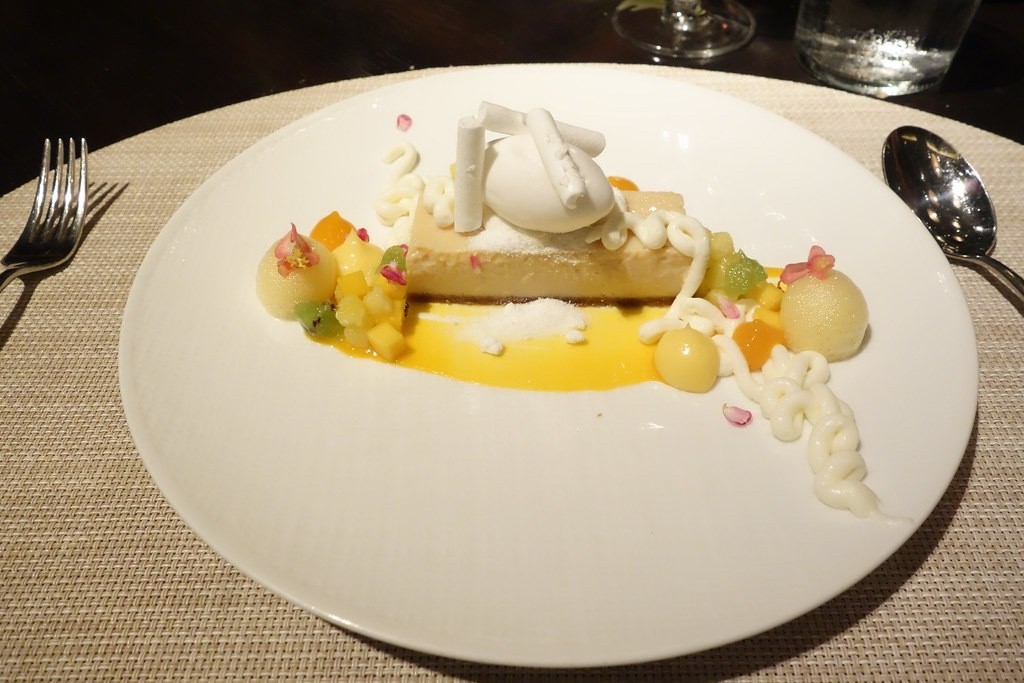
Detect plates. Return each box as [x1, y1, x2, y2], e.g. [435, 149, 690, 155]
[118, 64, 979, 668]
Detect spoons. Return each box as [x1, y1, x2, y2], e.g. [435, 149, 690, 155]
[879, 124, 1024, 307]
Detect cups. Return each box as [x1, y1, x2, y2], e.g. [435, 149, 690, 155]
[794, 0, 978, 97]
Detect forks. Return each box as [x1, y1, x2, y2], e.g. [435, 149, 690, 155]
[0, 136, 90, 292]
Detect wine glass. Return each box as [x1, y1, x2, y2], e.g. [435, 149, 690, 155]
[610, 0, 758, 56]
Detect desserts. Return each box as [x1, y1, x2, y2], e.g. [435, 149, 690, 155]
[242, 102, 879, 528]
